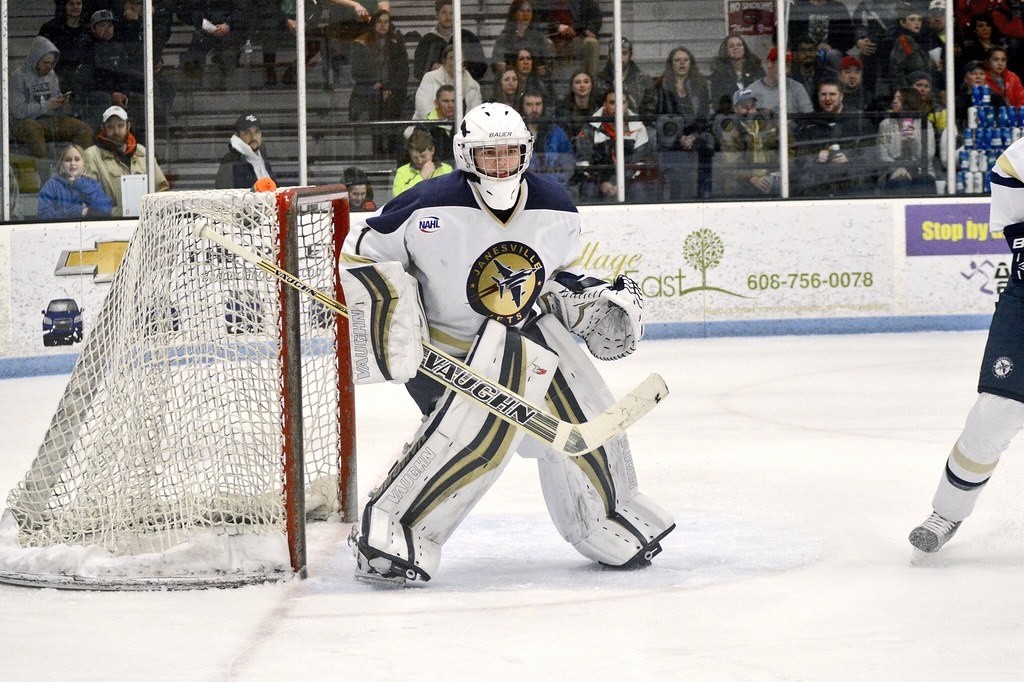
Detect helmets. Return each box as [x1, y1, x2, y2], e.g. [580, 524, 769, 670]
[453, 102, 533, 173]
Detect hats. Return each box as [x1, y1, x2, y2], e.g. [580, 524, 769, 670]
[732, 89, 758, 107]
[839, 56, 863, 69]
[928, 0, 946, 11]
[90, 10, 117, 25]
[964, 60, 987, 71]
[768, 47, 793, 61]
[102, 106, 128, 123]
[234, 112, 261, 133]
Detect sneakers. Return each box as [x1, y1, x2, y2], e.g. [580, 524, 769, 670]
[908, 509, 962, 567]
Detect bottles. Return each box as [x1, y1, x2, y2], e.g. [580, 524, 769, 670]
[955, 106, 1024, 195]
[245, 40, 252, 64]
[981, 83, 991, 106]
[971, 83, 982, 106]
[235, 178, 276, 229]
[818, 40, 826, 60]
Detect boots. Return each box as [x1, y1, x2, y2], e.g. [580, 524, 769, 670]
[372, 135, 401, 160]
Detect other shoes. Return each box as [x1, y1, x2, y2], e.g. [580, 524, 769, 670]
[261, 80, 279, 88]
[280, 79, 297, 88]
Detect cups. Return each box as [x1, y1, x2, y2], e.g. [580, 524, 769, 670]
[935, 181, 946, 195]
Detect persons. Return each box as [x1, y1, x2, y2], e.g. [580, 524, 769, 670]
[341, 167, 376, 211]
[339, 102, 677, 587]
[175, 0, 390, 89]
[9, 0, 176, 220]
[215, 113, 278, 189]
[908, 137, 1024, 553]
[350, 0, 1024, 204]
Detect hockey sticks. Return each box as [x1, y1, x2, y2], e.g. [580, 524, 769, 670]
[194, 219, 670, 458]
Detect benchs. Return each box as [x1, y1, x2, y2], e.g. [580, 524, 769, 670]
[9, 0, 1024, 199]
